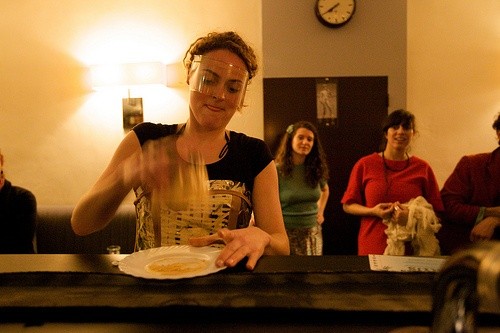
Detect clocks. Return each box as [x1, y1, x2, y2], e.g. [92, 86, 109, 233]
[315, 0, 356, 28]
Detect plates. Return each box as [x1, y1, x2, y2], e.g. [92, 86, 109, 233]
[117, 244, 227, 281]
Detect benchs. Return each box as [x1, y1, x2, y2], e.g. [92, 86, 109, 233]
[36, 203, 136, 255]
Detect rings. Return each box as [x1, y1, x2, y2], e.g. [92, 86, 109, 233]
[395, 206, 399, 210]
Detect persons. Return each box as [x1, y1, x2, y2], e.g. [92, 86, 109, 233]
[343, 109, 444, 257]
[70, 32, 289, 271]
[436, 114, 500, 257]
[275, 120, 330, 256]
[0, 153, 36, 255]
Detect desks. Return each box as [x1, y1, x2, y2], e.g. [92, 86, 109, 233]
[0, 248, 500, 333]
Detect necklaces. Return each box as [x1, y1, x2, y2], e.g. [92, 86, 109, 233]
[381, 150, 410, 172]
